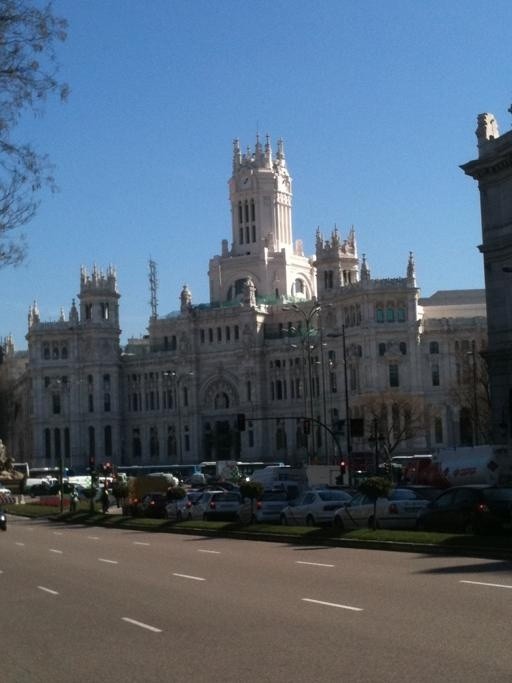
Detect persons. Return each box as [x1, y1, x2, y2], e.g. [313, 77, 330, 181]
[100, 490, 109, 514]
[69, 488, 79, 511]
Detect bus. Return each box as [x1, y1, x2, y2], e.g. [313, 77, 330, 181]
[116, 463, 200, 484]
[198, 459, 284, 482]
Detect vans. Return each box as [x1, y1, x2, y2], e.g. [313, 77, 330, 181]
[379, 454, 433, 475]
[251, 464, 310, 492]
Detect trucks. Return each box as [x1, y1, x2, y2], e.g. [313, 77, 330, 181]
[418, 443, 512, 485]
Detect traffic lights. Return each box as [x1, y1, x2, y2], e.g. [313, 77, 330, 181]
[340, 459, 346, 475]
[88, 455, 95, 469]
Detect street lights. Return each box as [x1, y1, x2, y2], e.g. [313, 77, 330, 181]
[163, 370, 194, 465]
[280, 302, 333, 467]
[326, 323, 353, 487]
